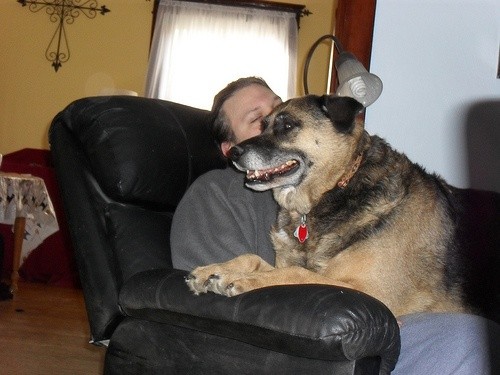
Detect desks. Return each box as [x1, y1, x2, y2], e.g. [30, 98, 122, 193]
[0, 172, 59, 297]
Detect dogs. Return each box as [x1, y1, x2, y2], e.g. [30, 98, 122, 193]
[184, 92, 500, 314]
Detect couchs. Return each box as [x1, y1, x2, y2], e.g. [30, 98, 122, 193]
[48, 96, 500, 375]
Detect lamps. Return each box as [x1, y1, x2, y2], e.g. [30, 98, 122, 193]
[303, 34, 383, 108]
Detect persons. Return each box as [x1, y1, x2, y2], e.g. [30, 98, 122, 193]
[171, 77, 500, 375]
